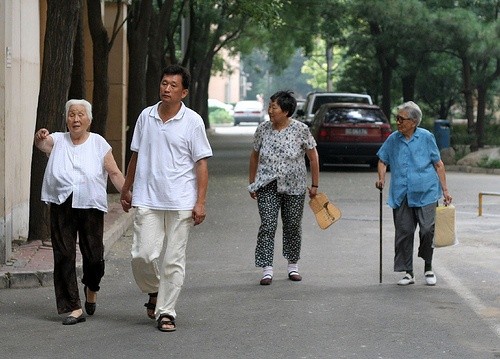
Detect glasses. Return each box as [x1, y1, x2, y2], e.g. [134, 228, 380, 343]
[396, 116, 410, 124]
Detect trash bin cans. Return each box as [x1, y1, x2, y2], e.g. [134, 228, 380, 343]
[433, 120, 450, 150]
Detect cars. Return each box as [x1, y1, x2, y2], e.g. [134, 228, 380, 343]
[233, 100, 266, 126]
[301, 102, 394, 172]
[208, 98, 233, 114]
[294, 98, 308, 116]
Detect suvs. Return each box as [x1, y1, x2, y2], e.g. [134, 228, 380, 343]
[296, 92, 374, 132]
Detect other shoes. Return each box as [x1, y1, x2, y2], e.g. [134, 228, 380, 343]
[84, 285, 97, 315]
[288, 271, 302, 281]
[62, 312, 86, 324]
[260, 274, 272, 285]
[424, 270, 437, 286]
[398, 273, 416, 285]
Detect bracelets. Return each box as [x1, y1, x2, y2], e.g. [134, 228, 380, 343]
[312, 185, 318, 187]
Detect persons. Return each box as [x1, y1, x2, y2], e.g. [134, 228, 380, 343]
[376, 101, 452, 285]
[120, 66, 213, 331]
[36, 99, 132, 324]
[247, 90, 319, 284]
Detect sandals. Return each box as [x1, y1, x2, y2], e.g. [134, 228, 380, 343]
[143, 292, 158, 319]
[157, 313, 176, 331]
